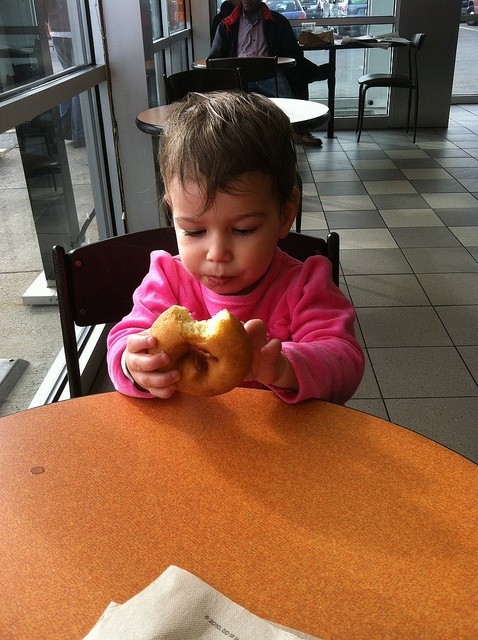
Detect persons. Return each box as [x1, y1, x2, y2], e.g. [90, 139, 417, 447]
[207, 0, 323, 148]
[106, 91, 366, 403]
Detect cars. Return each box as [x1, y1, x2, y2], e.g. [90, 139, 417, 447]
[461, 0, 478, 28]
[266, 0, 367, 37]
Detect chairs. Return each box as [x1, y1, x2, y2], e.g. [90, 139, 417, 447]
[161, 67, 247, 105]
[51, 226, 339, 400]
[204, 55, 279, 98]
[356, 31, 425, 144]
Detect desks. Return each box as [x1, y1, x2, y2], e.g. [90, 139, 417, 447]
[190, 55, 296, 76]
[0, 386, 478, 640]
[296, 36, 409, 139]
[136, 97, 332, 234]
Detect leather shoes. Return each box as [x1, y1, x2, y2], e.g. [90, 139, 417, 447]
[295, 132, 323, 147]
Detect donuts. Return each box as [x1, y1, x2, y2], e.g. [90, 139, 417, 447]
[146, 307, 254, 397]
